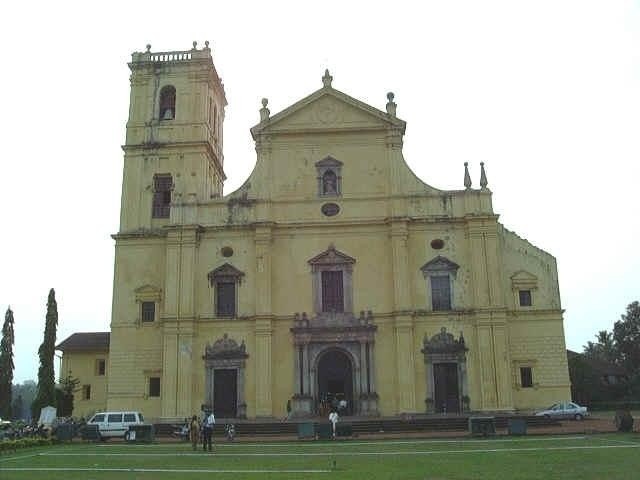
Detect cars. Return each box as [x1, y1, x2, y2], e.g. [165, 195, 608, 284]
[529, 400, 592, 423]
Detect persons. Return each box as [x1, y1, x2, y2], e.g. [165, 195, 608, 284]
[190, 415, 200, 451]
[328, 408, 340, 438]
[200, 413, 215, 452]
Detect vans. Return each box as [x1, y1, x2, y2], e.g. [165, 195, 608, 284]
[86, 410, 146, 442]
